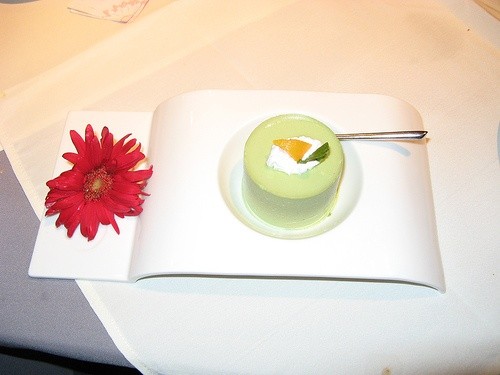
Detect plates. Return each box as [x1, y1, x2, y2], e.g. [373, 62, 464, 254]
[227, 150, 358, 239]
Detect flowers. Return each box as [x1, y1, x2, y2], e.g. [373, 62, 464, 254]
[43, 120, 155, 245]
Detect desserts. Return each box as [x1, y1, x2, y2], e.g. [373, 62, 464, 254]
[241, 113, 344, 226]
